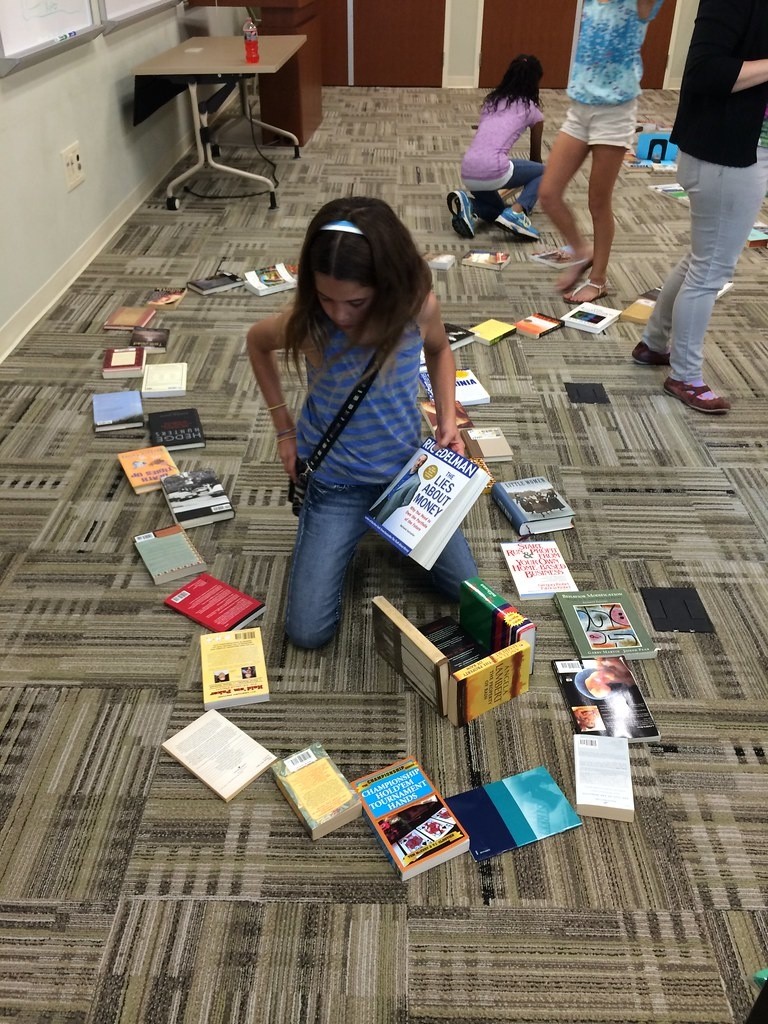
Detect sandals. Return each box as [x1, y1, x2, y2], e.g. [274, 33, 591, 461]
[564, 279, 612, 302]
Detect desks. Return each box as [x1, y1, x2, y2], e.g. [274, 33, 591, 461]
[134, 35, 307, 209]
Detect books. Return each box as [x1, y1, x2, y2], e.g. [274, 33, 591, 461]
[362, 436, 492, 571]
[187, 262, 298, 297]
[501, 541, 579, 601]
[443, 282, 735, 351]
[419, 245, 587, 272]
[746, 220, 768, 249]
[420, 346, 515, 463]
[93, 287, 236, 530]
[131, 523, 269, 711]
[623, 123, 679, 173]
[491, 476, 576, 537]
[649, 183, 691, 208]
[161, 709, 582, 882]
[551, 590, 661, 823]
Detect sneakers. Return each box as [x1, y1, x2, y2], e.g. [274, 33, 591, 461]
[445, 188, 477, 238]
[491, 205, 544, 242]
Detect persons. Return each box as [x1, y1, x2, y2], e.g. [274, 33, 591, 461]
[368, 454, 428, 524]
[538, 0, 653, 305]
[447, 53, 546, 240]
[515, 489, 565, 517]
[247, 196, 480, 650]
[631, 0, 768, 412]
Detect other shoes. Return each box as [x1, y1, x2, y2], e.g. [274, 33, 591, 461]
[662, 376, 732, 418]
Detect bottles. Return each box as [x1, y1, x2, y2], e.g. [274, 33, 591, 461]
[242, 16, 260, 63]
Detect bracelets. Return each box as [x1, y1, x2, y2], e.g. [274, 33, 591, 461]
[267, 402, 286, 411]
[276, 426, 297, 443]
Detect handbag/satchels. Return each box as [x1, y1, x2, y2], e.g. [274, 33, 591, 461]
[287, 456, 310, 516]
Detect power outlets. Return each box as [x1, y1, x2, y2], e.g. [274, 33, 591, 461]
[62, 142, 85, 192]
